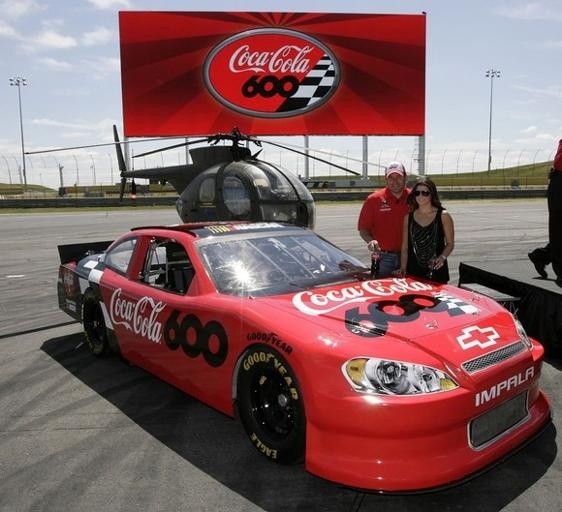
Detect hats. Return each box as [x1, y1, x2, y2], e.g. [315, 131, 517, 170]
[384, 163, 406, 178]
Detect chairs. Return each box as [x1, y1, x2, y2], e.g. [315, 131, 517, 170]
[167, 261, 194, 288]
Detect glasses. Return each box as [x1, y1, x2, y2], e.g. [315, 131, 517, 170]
[415, 191, 430, 196]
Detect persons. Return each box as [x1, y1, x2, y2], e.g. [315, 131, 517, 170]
[528, 137, 561, 287]
[358, 162, 418, 277]
[400, 178, 454, 286]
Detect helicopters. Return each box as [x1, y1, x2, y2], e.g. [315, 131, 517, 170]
[24, 124, 422, 232]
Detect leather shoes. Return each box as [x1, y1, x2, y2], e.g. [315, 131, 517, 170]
[528, 248, 548, 278]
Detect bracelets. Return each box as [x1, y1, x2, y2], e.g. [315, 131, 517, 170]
[440, 253, 446, 261]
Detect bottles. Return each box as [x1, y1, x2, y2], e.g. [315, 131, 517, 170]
[428, 255, 437, 272]
[371, 248, 380, 275]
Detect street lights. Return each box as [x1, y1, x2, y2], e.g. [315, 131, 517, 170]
[9, 72, 27, 188]
[485, 67, 500, 177]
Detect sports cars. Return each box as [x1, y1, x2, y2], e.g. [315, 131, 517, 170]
[58, 221, 553, 498]
